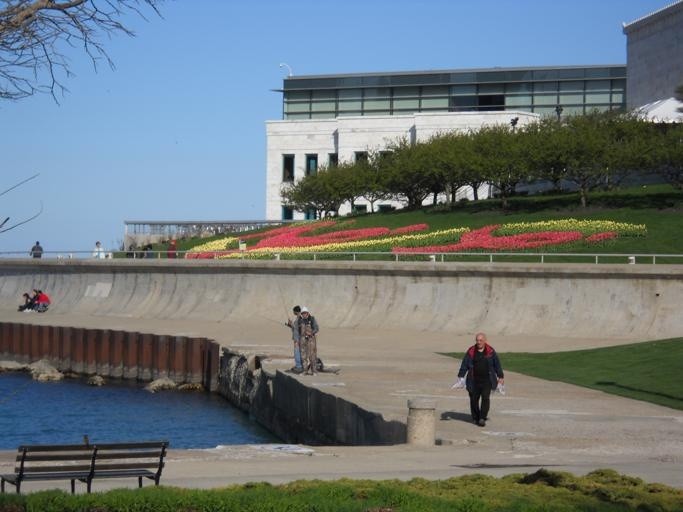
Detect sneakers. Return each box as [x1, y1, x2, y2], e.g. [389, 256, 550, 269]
[292, 368, 318, 376]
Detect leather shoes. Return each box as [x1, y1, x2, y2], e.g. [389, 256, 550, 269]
[473, 420, 479, 424]
[479, 419, 485, 426]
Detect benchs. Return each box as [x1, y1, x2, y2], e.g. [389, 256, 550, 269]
[0, 440, 170, 493]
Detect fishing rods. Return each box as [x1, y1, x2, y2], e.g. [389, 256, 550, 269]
[277, 285, 290, 320]
[262, 315, 288, 326]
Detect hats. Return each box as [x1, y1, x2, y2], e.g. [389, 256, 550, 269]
[301, 306, 309, 314]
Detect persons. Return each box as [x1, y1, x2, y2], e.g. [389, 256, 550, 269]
[458, 333, 503, 427]
[126, 240, 176, 258]
[93, 241, 105, 259]
[30, 241, 44, 259]
[18, 288, 52, 312]
[288, 306, 319, 376]
